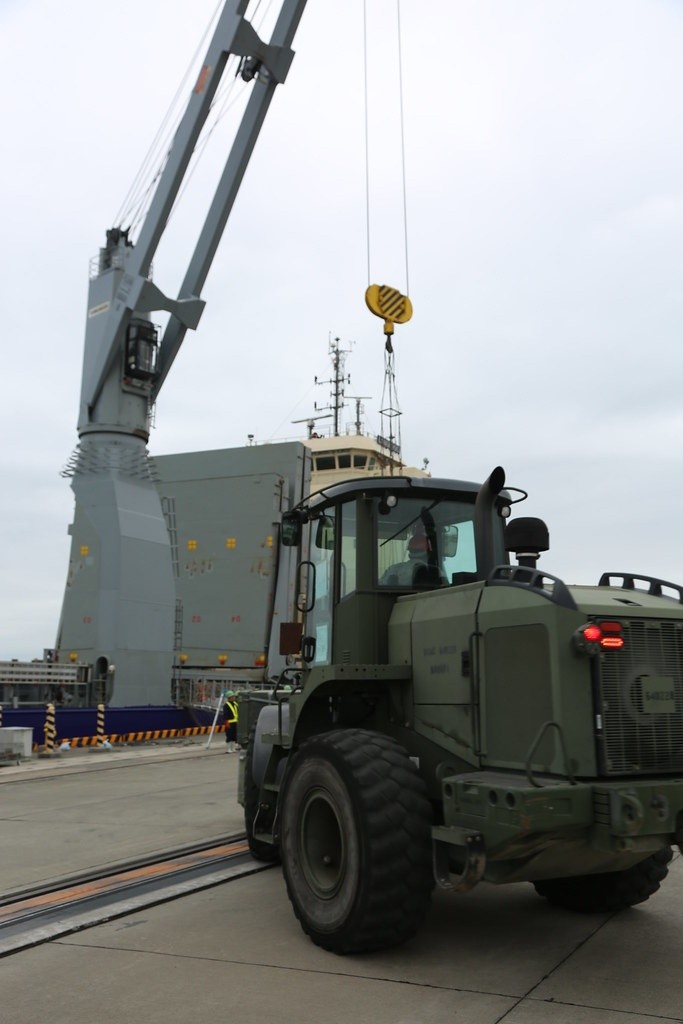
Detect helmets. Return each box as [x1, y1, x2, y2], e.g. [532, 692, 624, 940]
[406, 534, 428, 551]
[234, 691, 240, 696]
[226, 690, 234, 698]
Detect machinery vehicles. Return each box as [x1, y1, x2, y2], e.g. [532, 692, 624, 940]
[235, 464, 682, 956]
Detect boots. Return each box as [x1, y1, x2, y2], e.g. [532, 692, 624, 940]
[225, 741, 237, 753]
[235, 743, 242, 750]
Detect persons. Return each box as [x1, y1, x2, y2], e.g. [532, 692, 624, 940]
[378, 533, 431, 586]
[223, 689, 242, 753]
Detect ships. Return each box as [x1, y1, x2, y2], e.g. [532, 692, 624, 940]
[0, 330, 414, 754]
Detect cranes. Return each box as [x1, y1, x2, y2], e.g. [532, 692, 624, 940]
[47, 1, 416, 710]
